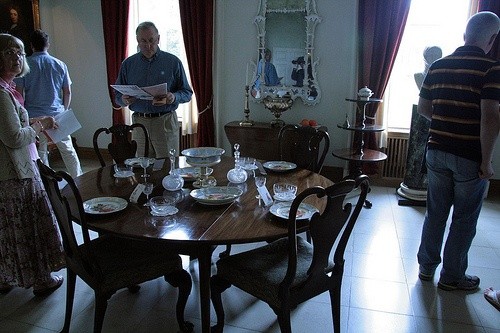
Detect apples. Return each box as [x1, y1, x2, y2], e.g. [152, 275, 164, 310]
[302, 119, 317, 126]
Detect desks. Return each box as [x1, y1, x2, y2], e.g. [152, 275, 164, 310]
[59, 156, 345, 333]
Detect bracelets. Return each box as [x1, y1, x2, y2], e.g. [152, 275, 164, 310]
[38, 121, 44, 132]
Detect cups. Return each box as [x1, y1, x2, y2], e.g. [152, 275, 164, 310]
[236, 156, 256, 179]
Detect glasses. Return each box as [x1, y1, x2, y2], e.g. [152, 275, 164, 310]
[138, 40, 159, 48]
[2, 50, 26, 57]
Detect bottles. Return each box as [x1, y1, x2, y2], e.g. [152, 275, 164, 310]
[162, 146, 185, 192]
[228, 182, 248, 217]
[227, 144, 248, 184]
[163, 189, 185, 209]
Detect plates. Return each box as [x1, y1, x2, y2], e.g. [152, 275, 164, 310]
[189, 185, 243, 206]
[83, 197, 128, 214]
[169, 166, 213, 181]
[269, 202, 320, 220]
[264, 160, 297, 171]
[124, 157, 156, 166]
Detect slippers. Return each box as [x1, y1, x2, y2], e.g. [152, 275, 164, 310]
[484, 290, 500, 310]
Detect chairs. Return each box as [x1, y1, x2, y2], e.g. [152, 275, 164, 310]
[206, 174, 370, 333]
[34, 159, 195, 333]
[93, 123, 150, 167]
[278, 124, 331, 173]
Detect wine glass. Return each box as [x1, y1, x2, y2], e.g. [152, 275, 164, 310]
[254, 176, 266, 198]
[139, 158, 150, 178]
[142, 182, 154, 207]
[255, 198, 266, 218]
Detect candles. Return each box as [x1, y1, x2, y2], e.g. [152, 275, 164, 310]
[246, 63, 248, 85]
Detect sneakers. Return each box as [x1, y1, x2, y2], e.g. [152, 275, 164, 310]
[439, 274, 481, 291]
[419, 272, 435, 281]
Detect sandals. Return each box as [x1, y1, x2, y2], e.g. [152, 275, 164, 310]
[0, 283, 15, 294]
[32, 274, 64, 297]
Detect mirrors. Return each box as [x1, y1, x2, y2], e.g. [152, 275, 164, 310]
[253, 0, 321, 106]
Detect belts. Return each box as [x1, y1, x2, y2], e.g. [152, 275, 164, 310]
[134, 110, 173, 118]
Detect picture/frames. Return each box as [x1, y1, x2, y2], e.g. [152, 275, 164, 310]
[0, 0, 41, 54]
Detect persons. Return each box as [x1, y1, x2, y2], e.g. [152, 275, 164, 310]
[0, 33, 64, 297]
[113, 21, 192, 159]
[13, 29, 83, 178]
[416, 11, 500, 312]
[259, 48, 281, 85]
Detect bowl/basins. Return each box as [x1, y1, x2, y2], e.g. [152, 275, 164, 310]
[149, 196, 176, 214]
[273, 183, 297, 201]
[180, 147, 226, 164]
[114, 164, 135, 177]
[145, 215, 176, 228]
[115, 178, 137, 188]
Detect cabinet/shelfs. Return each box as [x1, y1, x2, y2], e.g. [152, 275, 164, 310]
[224, 120, 319, 169]
[332, 98, 387, 207]
